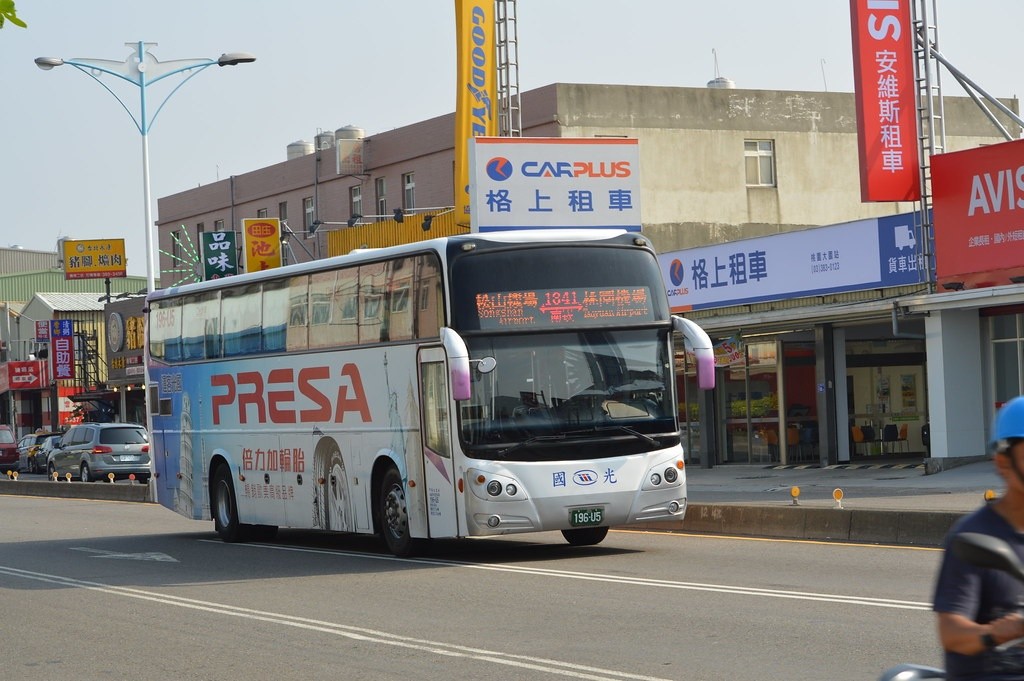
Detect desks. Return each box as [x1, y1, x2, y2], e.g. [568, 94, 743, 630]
[848, 410, 924, 455]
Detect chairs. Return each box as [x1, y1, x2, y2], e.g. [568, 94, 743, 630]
[851, 424, 912, 456]
[761, 426, 817, 463]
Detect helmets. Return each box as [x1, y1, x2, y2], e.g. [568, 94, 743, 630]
[993, 396, 1023, 439]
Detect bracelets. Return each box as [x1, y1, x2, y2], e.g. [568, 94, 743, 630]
[978, 633, 999, 648]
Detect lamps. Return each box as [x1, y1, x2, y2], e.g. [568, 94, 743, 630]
[279, 205, 456, 245]
[1008, 275, 1024, 284]
[942, 282, 965, 291]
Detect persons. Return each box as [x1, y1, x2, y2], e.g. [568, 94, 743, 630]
[933, 397, 1024, 681]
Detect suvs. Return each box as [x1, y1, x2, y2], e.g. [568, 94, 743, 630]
[47, 422, 151, 484]
[0, 424, 20, 474]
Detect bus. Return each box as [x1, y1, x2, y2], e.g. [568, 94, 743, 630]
[145, 229, 714, 559]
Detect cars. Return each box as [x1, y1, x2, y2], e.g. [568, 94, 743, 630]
[13, 433, 62, 473]
[34, 436, 61, 474]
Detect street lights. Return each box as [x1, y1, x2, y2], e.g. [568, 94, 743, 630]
[34, 41, 257, 503]
